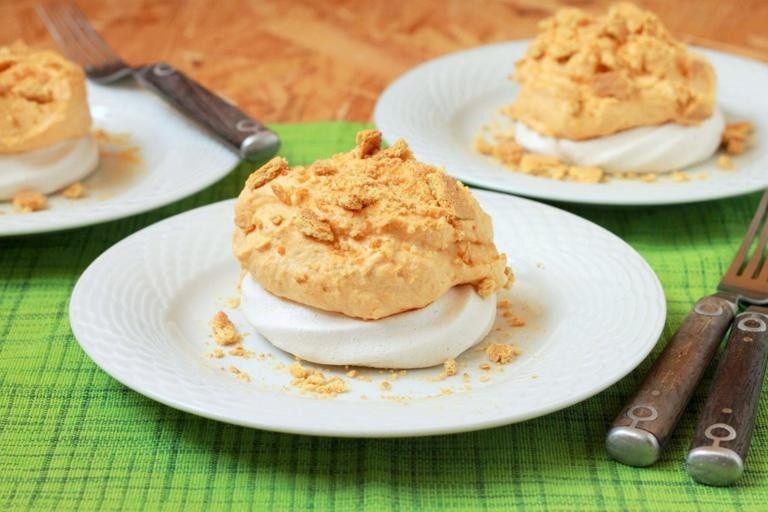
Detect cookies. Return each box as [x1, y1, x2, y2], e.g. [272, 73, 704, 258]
[229, 127, 516, 322]
[499, 1, 717, 140]
[0, 39, 98, 156]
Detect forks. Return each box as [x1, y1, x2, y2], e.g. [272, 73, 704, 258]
[606, 188, 767, 488]
[37, 1, 284, 160]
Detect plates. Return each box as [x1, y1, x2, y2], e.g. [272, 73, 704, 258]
[0, 82, 245, 239]
[376, 39, 765, 207]
[67, 188, 669, 446]
